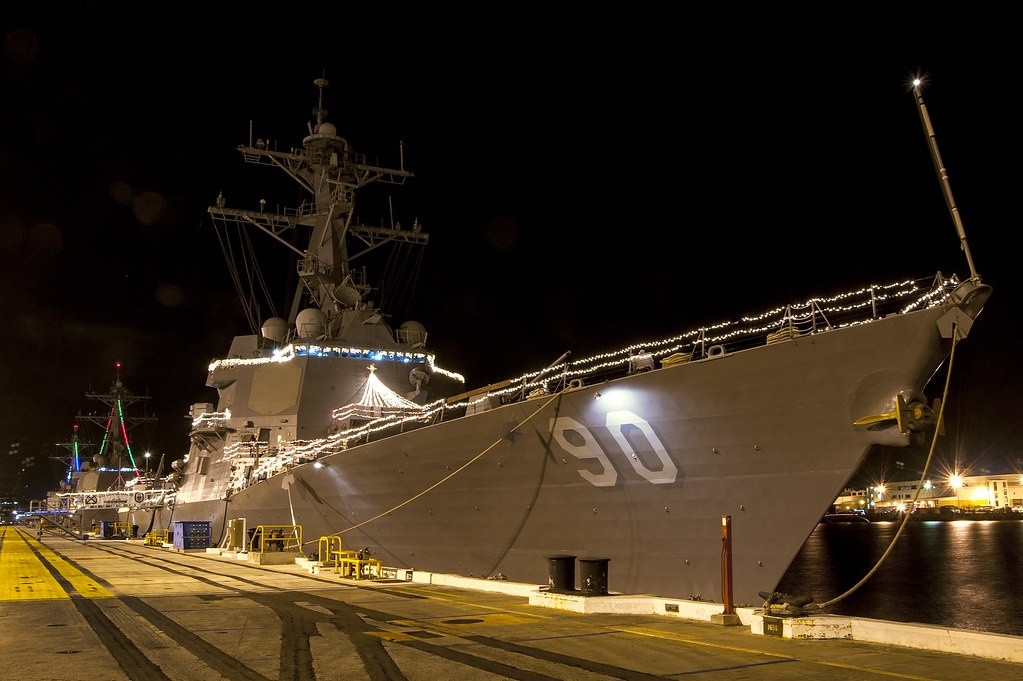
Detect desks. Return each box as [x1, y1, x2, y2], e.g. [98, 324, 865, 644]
[381, 567, 397, 579]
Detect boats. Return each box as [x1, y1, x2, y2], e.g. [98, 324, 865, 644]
[43, 77, 993, 614]
